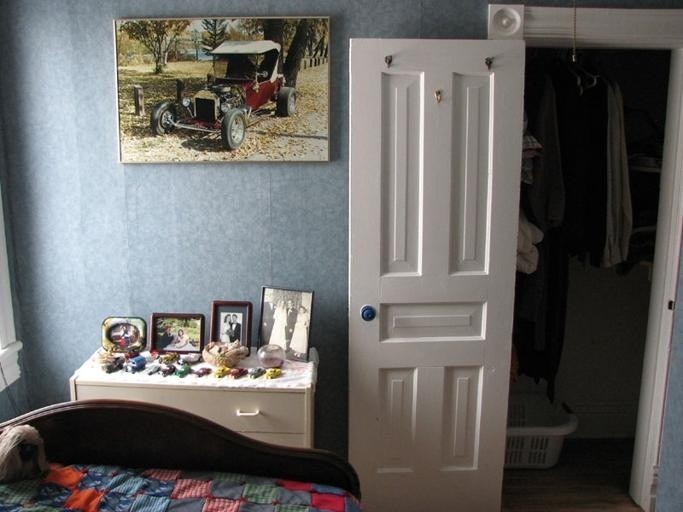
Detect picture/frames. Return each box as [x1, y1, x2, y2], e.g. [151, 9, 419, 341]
[100, 283, 314, 363]
[110, 16, 332, 164]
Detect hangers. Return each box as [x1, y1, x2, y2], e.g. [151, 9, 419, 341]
[564, 47, 597, 96]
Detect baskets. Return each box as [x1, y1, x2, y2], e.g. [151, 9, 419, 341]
[503, 391, 579, 469]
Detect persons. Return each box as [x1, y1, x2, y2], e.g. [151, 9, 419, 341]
[120, 324, 130, 347]
[221, 314, 231, 341]
[230, 314, 240, 341]
[291, 306, 307, 358]
[269, 300, 286, 350]
[261, 296, 274, 344]
[285, 299, 297, 348]
[169, 329, 194, 350]
[161, 327, 173, 347]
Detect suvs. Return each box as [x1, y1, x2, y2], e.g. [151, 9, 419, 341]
[150, 38, 298, 153]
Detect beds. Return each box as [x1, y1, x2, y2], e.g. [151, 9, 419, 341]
[0, 397, 362, 512]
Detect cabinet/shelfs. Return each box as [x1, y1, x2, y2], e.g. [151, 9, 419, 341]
[68, 345, 316, 449]
